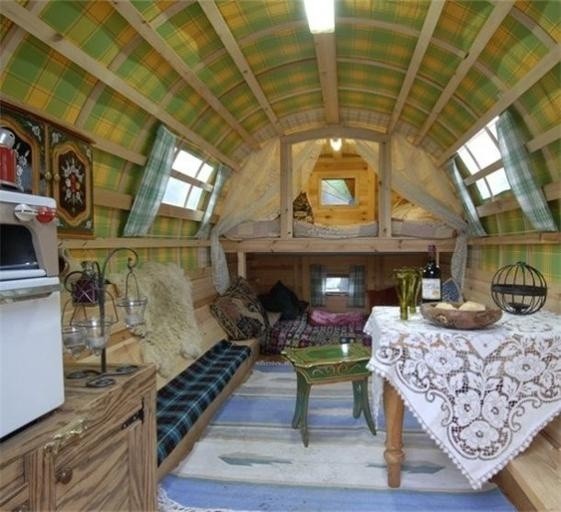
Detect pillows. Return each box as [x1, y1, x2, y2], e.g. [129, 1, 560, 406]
[391, 198, 463, 222]
[211, 275, 266, 340]
[258, 279, 310, 320]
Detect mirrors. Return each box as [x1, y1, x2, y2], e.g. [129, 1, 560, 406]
[308, 263, 366, 308]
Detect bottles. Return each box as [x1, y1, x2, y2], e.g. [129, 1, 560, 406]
[421, 245, 442, 303]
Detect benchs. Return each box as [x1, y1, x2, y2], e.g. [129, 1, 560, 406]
[76, 266, 260, 482]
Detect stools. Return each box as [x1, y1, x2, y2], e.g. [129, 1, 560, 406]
[281, 343, 376, 448]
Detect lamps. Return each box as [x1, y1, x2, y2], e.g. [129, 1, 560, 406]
[61, 247, 147, 388]
[329, 138, 342, 150]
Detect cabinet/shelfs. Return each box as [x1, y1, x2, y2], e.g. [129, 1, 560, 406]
[0, 364, 158, 512]
[0, 99, 96, 240]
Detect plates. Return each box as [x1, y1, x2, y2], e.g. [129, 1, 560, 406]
[421, 302, 502, 330]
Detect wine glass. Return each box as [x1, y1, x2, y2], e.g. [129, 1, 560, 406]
[391, 267, 422, 321]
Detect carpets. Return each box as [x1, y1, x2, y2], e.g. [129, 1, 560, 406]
[158, 360, 520, 512]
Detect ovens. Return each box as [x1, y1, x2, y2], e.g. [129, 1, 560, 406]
[0, 189, 65, 439]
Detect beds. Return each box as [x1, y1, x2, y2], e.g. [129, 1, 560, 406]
[261, 312, 371, 356]
[219, 215, 457, 253]
